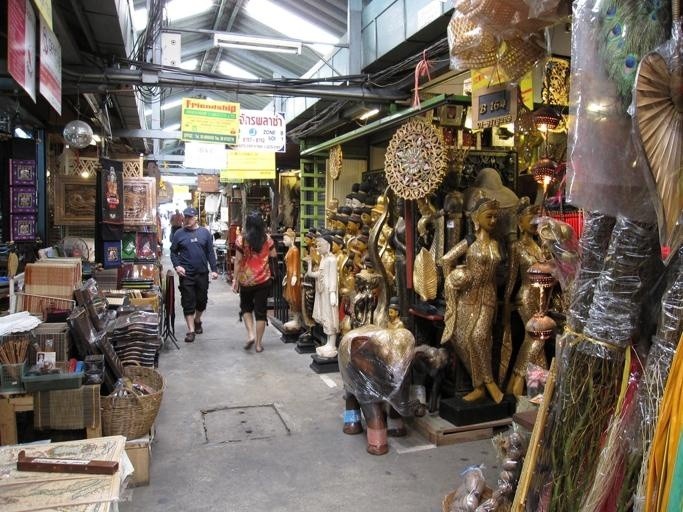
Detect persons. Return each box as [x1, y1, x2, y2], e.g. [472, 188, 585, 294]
[230, 212, 277, 352]
[170, 206, 219, 343]
[278, 183, 555, 403]
[158, 209, 184, 250]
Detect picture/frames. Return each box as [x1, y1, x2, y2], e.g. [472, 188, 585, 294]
[54, 175, 156, 225]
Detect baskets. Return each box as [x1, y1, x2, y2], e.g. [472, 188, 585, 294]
[100, 360, 164, 438]
[449, 1, 554, 82]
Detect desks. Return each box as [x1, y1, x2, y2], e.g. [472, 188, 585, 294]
[161, 270, 179, 350]
[0, 435, 134, 512]
[0, 384, 101, 445]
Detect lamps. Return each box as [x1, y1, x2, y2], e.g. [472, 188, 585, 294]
[64, 83, 92, 148]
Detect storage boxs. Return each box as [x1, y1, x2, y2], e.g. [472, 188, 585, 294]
[124, 431, 149, 488]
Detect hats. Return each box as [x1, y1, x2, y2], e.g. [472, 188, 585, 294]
[183, 207, 198, 216]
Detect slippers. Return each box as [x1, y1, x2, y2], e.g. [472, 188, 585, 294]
[256, 346, 263, 352]
[244, 339, 255, 348]
[184, 332, 196, 342]
[194, 319, 203, 333]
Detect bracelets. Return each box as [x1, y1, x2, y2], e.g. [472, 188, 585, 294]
[232, 279, 237, 282]
